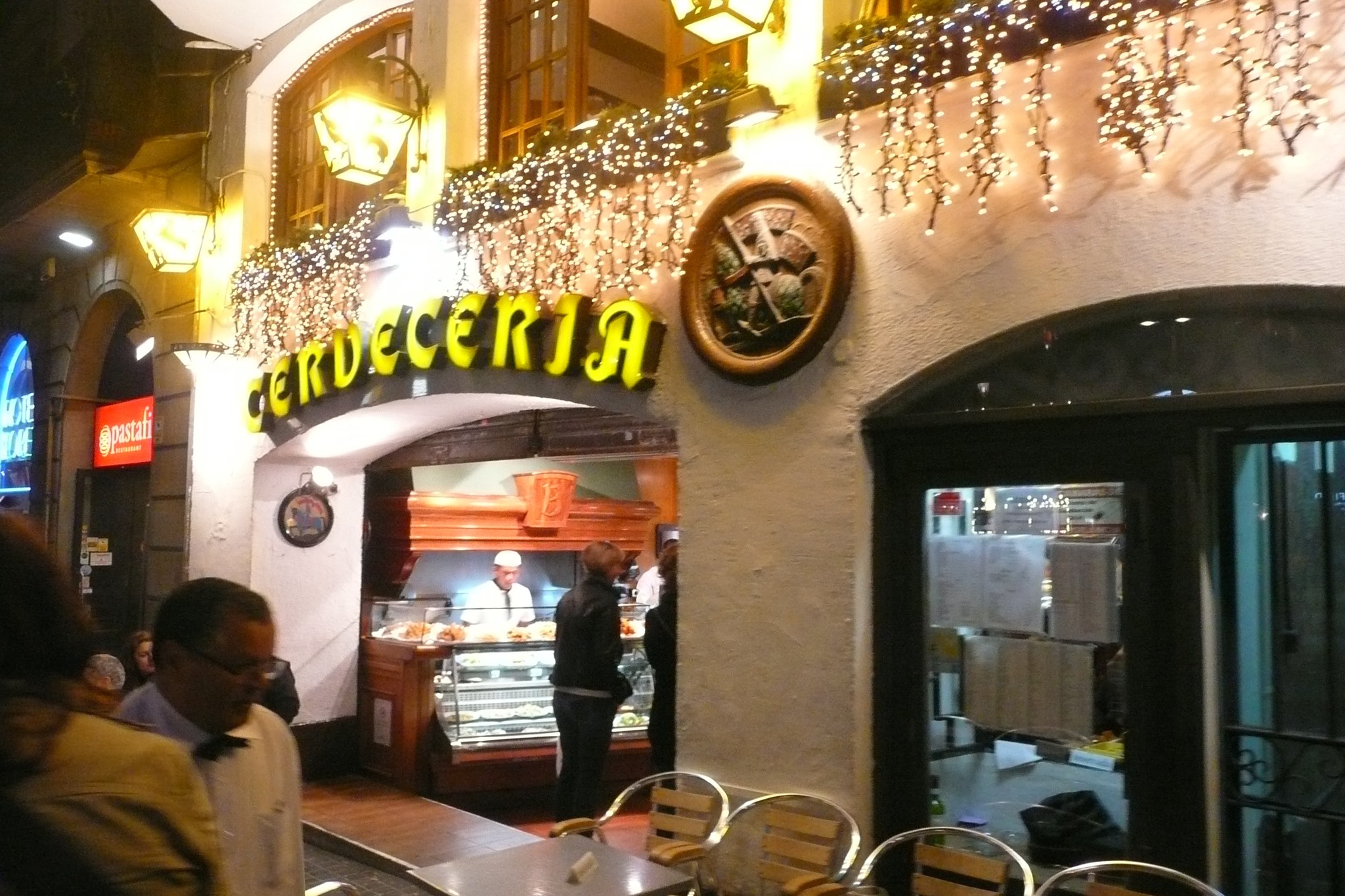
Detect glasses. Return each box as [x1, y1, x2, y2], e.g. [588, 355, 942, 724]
[184, 642, 289, 683]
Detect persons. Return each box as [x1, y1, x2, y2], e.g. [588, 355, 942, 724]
[547, 540, 635, 838]
[68, 614, 301, 738]
[459, 548, 539, 636]
[0, 505, 239, 896]
[643, 540, 679, 845]
[633, 538, 681, 620]
[109, 577, 307, 892]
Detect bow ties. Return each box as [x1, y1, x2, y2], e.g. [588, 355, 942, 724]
[195, 733, 249, 765]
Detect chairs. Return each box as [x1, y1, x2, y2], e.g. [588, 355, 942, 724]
[546, 759, 733, 878]
[688, 782, 864, 894]
[1030, 855, 1225, 896]
[789, 826, 1033, 896]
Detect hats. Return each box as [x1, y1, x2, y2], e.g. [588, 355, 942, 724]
[493, 549, 523, 569]
[660, 529, 678, 544]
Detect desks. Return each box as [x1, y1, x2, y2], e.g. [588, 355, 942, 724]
[400, 835, 691, 895]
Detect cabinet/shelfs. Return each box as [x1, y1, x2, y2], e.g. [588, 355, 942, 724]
[364, 592, 654, 800]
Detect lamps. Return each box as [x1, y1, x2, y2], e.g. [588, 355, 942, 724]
[669, 1, 796, 45]
[304, 52, 434, 193]
[124, 327, 158, 362]
[296, 466, 338, 499]
[124, 170, 218, 273]
